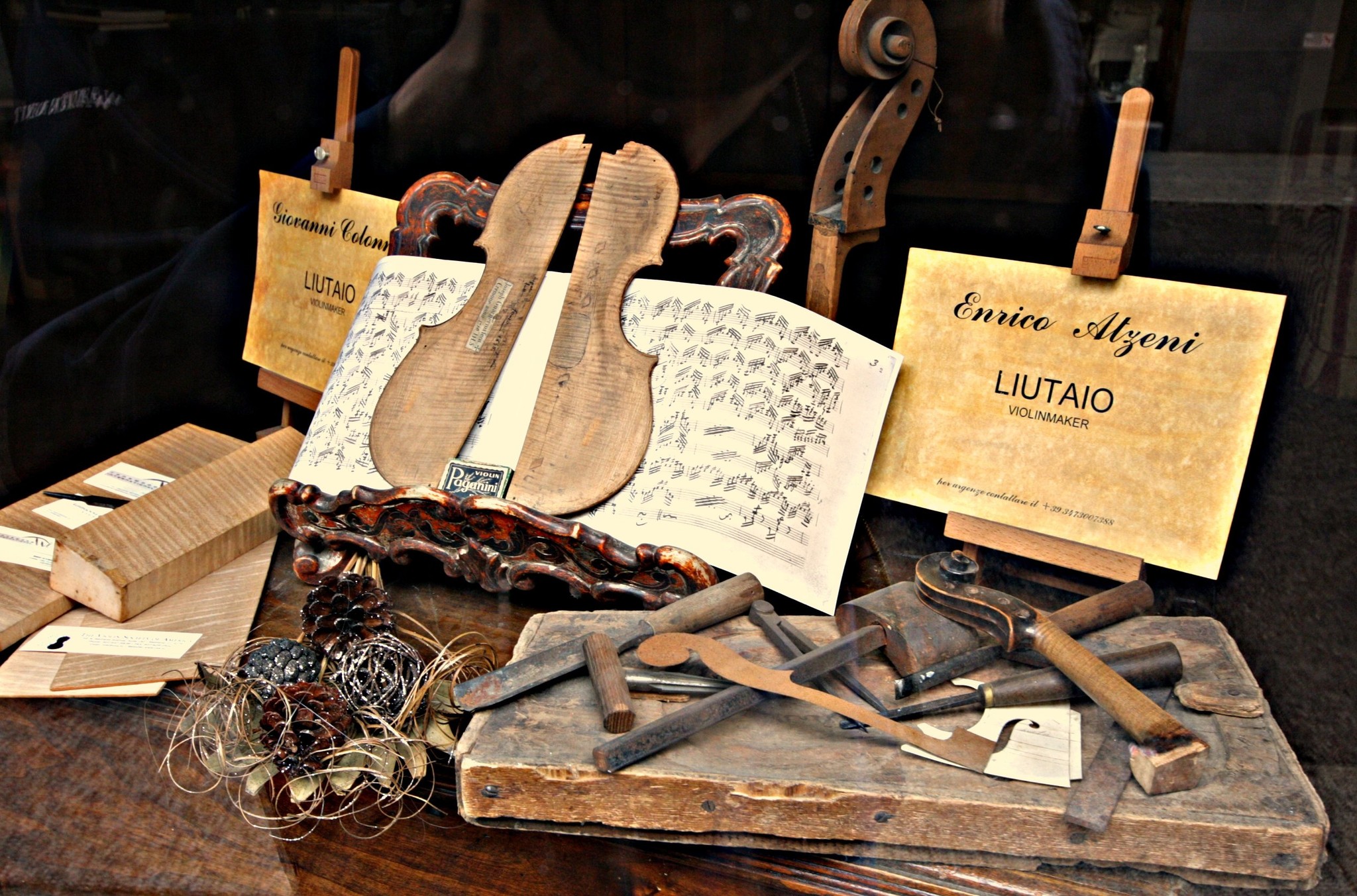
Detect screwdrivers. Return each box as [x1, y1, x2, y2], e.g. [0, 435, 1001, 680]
[840, 639, 1186, 733]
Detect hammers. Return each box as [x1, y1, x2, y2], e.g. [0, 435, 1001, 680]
[584, 631, 730, 735]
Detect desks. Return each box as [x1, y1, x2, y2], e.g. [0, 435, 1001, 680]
[0, 534, 1198, 896]
